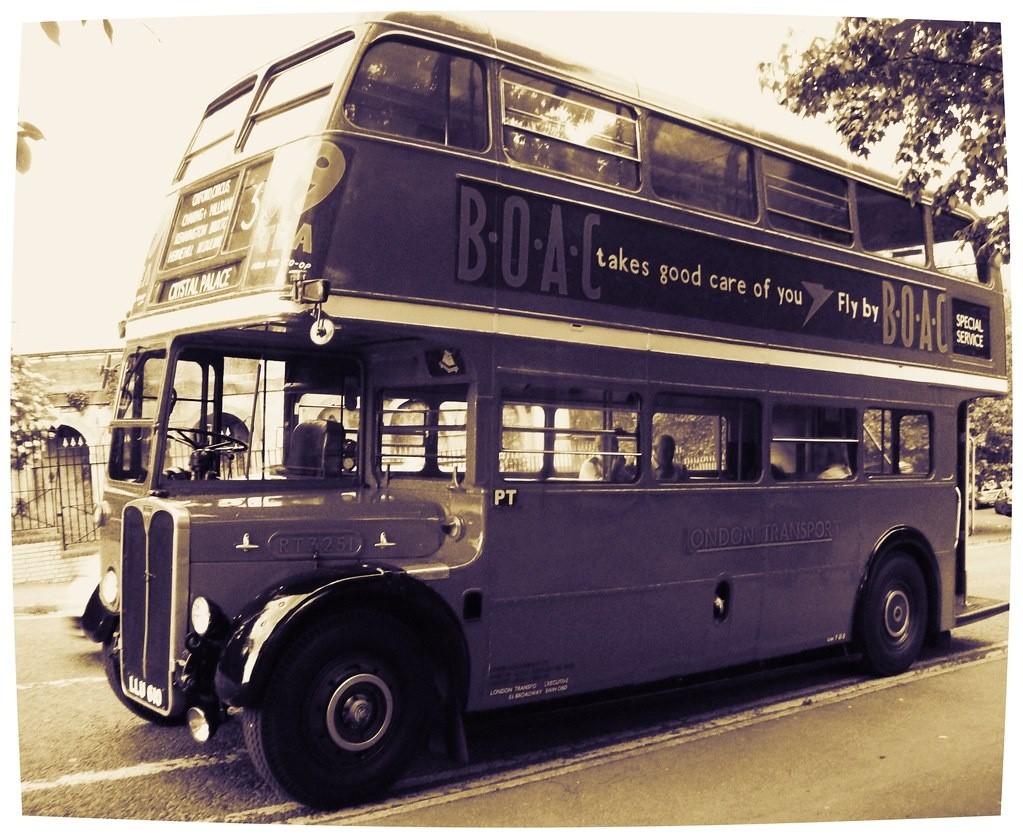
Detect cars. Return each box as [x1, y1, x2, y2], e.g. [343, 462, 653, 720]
[969, 471, 1012, 516]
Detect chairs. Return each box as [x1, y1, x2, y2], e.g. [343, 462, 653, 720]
[282, 420, 346, 478]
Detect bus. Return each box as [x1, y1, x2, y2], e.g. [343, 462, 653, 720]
[80, 12, 1010, 810]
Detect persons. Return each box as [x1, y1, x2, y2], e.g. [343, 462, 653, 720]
[814, 432, 853, 480]
[652, 433, 690, 482]
[579, 435, 636, 482]
